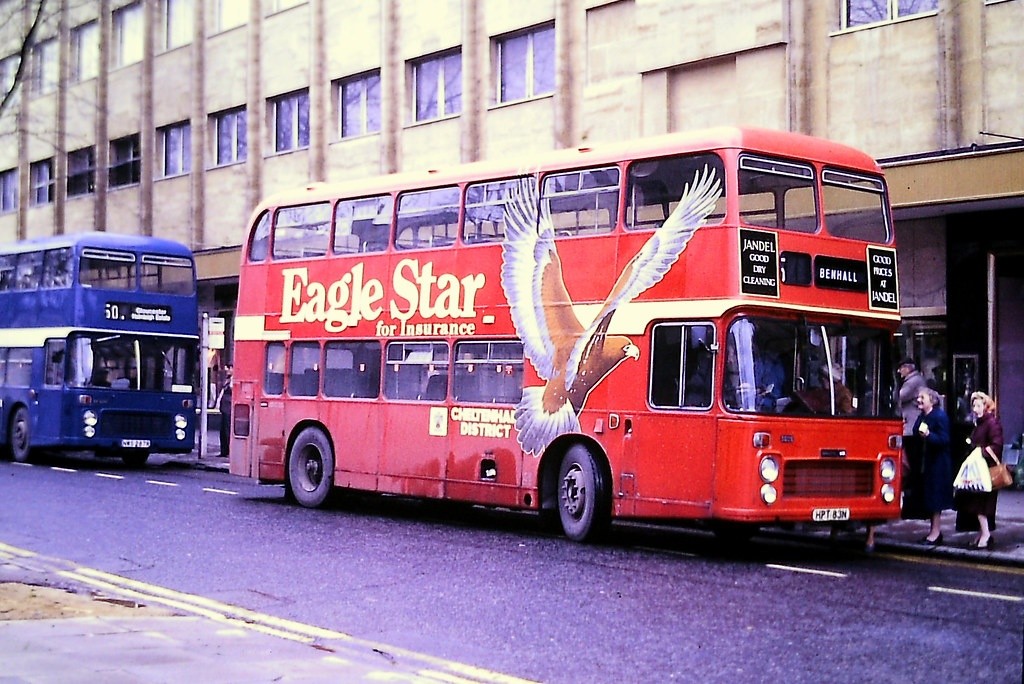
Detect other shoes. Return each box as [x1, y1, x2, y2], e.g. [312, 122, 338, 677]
[975, 536, 993, 550]
[919, 532, 943, 545]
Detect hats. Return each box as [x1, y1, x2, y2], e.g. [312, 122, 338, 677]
[895, 358, 913, 368]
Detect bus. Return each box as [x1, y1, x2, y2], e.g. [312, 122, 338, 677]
[228, 125, 903, 559]
[0, 230, 201, 468]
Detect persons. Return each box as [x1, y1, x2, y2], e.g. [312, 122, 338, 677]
[94, 368, 112, 388]
[779, 386, 828, 416]
[954, 391, 1004, 551]
[128, 366, 138, 388]
[216, 360, 234, 458]
[902, 387, 953, 545]
[894, 360, 928, 470]
[836, 385, 858, 415]
[208, 363, 219, 408]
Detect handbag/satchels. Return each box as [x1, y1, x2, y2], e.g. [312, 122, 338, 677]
[985, 446, 1012, 491]
[953, 447, 992, 492]
